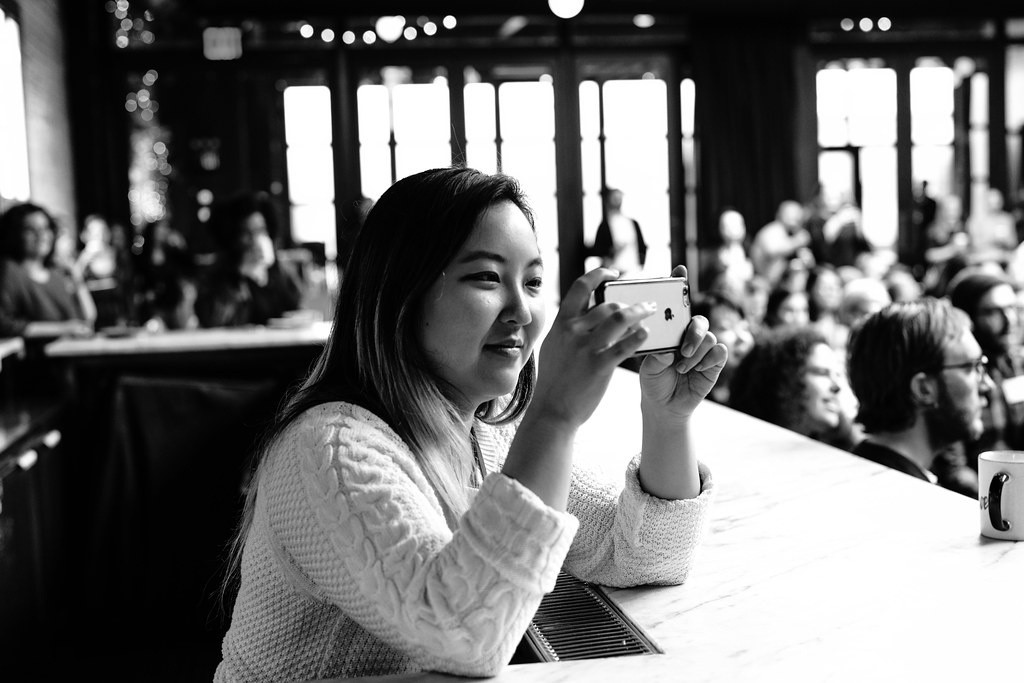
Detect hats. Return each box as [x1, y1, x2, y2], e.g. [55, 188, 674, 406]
[950, 263, 1008, 312]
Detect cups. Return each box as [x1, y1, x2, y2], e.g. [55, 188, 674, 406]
[977, 450, 1024, 541]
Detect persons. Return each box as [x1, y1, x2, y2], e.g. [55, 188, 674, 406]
[0, 203, 95, 346]
[212, 167, 729, 683]
[221, 210, 274, 324]
[699, 184, 1023, 498]
[75, 216, 131, 325]
[593, 191, 648, 300]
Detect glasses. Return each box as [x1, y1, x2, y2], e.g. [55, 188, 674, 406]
[939, 355, 992, 374]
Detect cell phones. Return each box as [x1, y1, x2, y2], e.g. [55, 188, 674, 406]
[594, 276, 692, 357]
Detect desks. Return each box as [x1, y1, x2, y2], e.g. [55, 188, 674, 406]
[44, 321, 332, 359]
[308, 345, 1024, 683]
